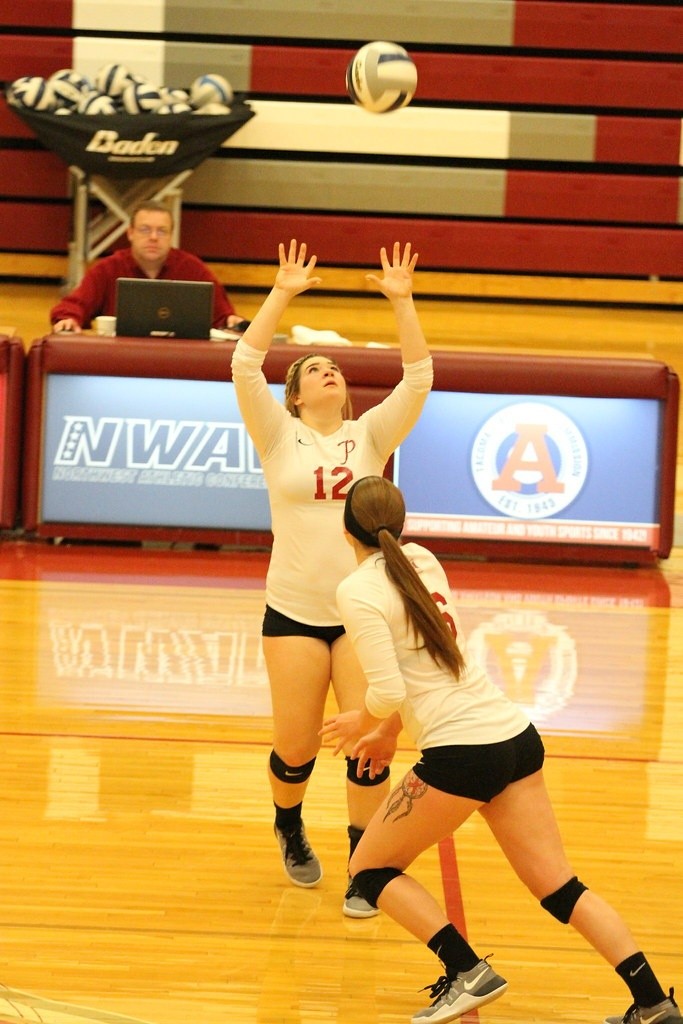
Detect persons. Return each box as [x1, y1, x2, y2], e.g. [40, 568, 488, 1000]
[50, 201, 244, 334]
[319, 475, 683, 1024]
[230, 238, 433, 920]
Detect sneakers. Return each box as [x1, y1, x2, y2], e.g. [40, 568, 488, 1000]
[343, 861, 381, 918]
[604, 987, 681, 1024]
[274, 817, 322, 888]
[411, 953, 508, 1024]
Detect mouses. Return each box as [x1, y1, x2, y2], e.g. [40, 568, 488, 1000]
[59, 328, 75, 334]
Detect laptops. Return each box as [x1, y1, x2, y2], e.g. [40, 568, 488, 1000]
[116, 279, 213, 340]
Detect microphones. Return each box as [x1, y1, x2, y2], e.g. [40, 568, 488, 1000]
[220, 320, 251, 332]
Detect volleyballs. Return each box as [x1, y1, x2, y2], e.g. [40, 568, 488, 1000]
[189, 73, 235, 110]
[47, 68, 119, 115]
[122, 80, 195, 129]
[194, 102, 232, 122]
[6, 74, 57, 114]
[345, 40, 419, 116]
[86, 62, 147, 114]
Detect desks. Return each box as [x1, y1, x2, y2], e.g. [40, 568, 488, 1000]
[0, 324, 681, 570]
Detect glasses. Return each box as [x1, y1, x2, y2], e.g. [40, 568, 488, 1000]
[132, 225, 171, 236]
